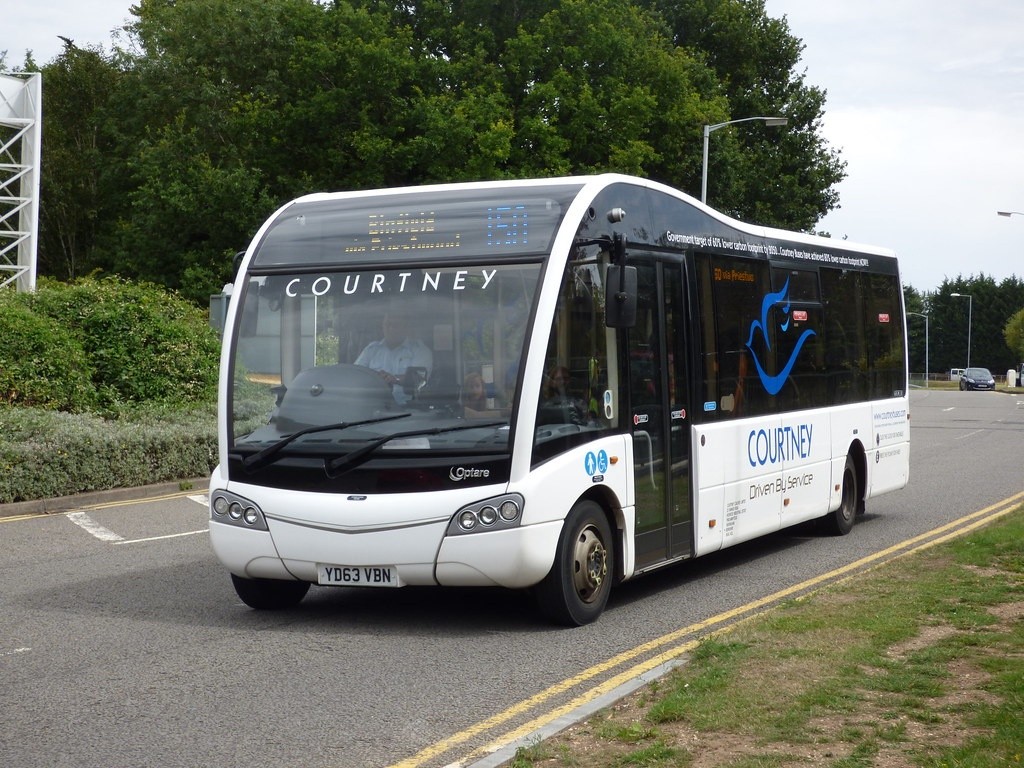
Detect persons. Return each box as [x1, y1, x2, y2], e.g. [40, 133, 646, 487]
[355, 306, 433, 406]
[458, 373, 512, 419]
[506, 335, 544, 416]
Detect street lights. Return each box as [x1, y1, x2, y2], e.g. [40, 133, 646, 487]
[997, 210, 1024, 216]
[907, 312, 930, 387]
[699, 114, 792, 205]
[950, 292, 973, 369]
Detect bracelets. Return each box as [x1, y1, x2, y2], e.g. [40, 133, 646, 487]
[395, 375, 400, 385]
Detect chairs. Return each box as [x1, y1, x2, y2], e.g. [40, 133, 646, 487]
[388, 360, 904, 426]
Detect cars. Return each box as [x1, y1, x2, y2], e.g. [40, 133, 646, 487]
[959, 368, 996, 391]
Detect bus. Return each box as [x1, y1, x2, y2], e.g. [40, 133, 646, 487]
[207, 171, 912, 627]
